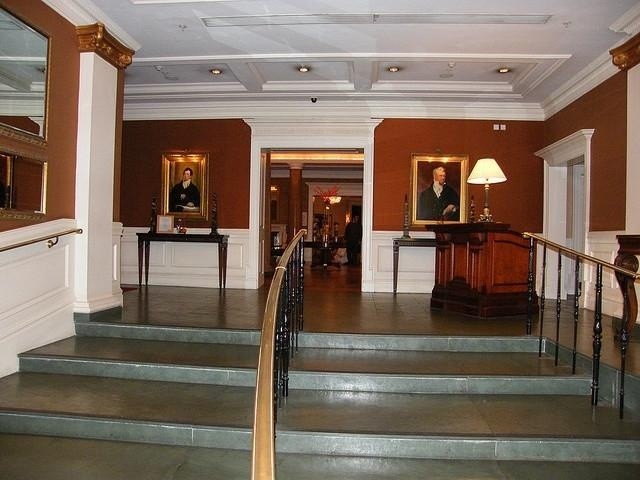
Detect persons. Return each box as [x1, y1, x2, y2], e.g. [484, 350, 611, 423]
[169, 166, 199, 212]
[419, 166, 460, 221]
[343, 213, 361, 265]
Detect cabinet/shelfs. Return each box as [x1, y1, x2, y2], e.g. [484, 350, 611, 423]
[424, 223, 540, 318]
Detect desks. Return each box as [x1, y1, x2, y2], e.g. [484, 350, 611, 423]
[390, 236, 437, 297]
[613, 234, 639, 343]
[134, 233, 230, 294]
[304, 241, 347, 271]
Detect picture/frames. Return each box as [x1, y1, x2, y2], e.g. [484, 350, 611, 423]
[159, 147, 211, 220]
[156, 214, 175, 234]
[409, 151, 468, 226]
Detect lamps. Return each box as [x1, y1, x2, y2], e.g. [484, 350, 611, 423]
[466, 157, 509, 222]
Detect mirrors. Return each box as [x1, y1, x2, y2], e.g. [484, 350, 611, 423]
[0, 143, 49, 223]
[0, 2, 55, 151]
